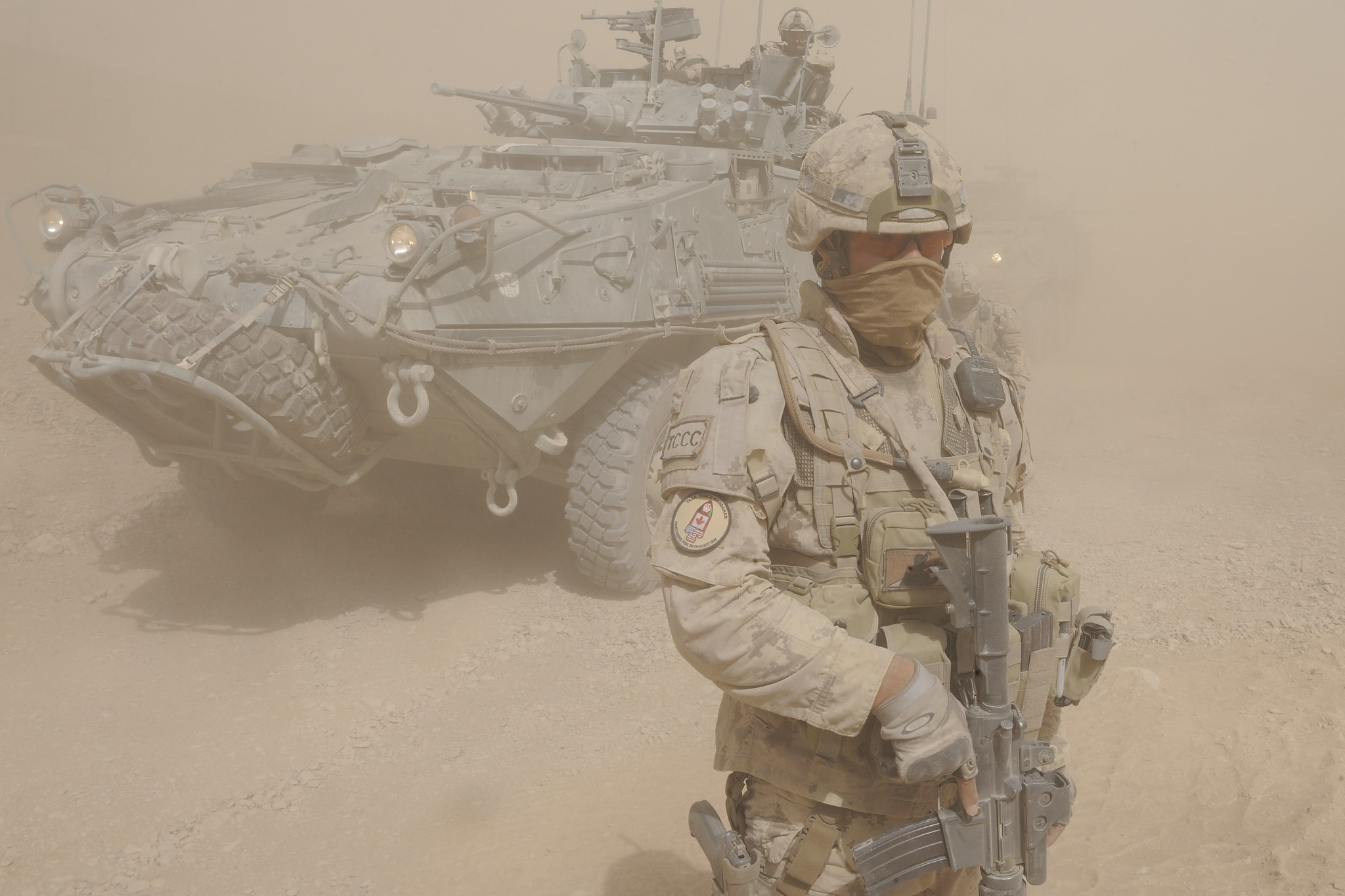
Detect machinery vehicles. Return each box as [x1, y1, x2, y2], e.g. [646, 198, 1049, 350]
[18, 9, 859, 598]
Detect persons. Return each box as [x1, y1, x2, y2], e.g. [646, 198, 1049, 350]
[657, 108, 1033, 895]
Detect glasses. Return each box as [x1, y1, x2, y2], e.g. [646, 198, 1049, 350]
[837, 229, 954, 259]
[782, 30, 812, 43]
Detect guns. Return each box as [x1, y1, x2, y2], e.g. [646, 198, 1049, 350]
[688, 800, 751, 893]
[848, 514, 1081, 896]
[582, 6, 703, 44]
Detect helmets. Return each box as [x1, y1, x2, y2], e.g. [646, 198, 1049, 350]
[778, 7, 815, 36]
[786, 111, 973, 252]
